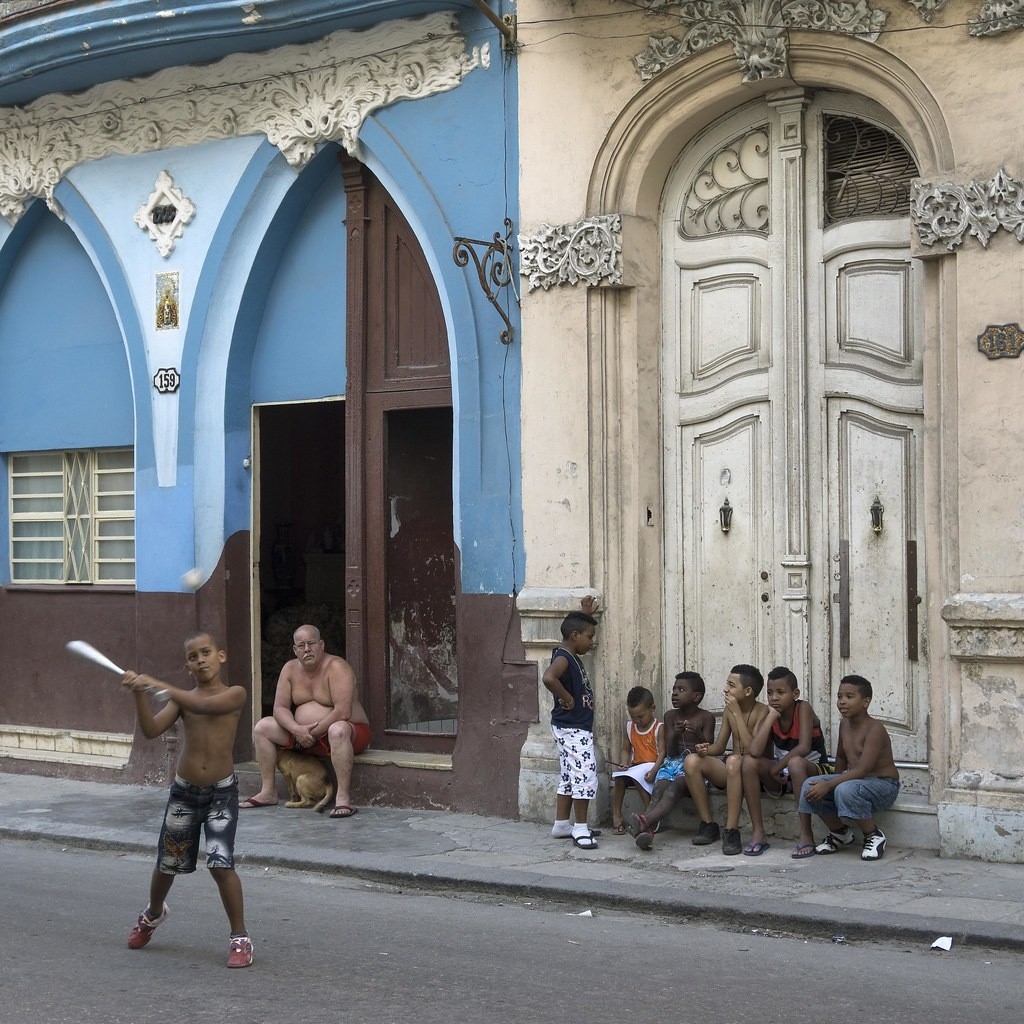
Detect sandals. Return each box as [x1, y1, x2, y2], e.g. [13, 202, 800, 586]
[629, 812, 648, 838]
[637, 830, 654, 851]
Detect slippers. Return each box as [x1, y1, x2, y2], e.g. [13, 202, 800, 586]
[238, 798, 278, 808]
[329, 806, 357, 818]
[612, 824, 627, 835]
[573, 833, 597, 849]
[743, 840, 770, 856]
[557, 828, 601, 836]
[653, 821, 660, 832]
[791, 842, 817, 858]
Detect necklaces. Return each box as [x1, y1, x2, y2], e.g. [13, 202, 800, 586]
[561, 647, 592, 691]
[736, 700, 756, 756]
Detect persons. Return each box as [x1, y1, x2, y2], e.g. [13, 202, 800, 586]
[741, 664, 827, 860]
[237, 620, 372, 818]
[543, 593, 603, 849]
[607, 685, 664, 834]
[120, 631, 255, 969]
[627, 671, 717, 851]
[683, 662, 771, 855]
[795, 672, 901, 862]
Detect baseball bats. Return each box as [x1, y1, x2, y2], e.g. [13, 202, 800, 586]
[64, 640, 171, 701]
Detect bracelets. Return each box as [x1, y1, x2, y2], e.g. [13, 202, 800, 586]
[568, 696, 574, 705]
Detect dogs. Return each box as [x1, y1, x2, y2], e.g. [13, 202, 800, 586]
[274, 747, 334, 811]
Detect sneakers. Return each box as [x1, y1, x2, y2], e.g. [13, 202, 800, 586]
[692, 821, 720, 845]
[227, 933, 254, 968]
[861, 826, 886, 860]
[722, 828, 742, 855]
[816, 826, 855, 855]
[128, 900, 169, 949]
